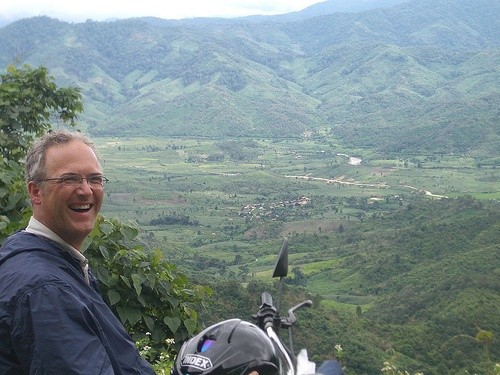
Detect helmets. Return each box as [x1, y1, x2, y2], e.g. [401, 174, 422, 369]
[170, 318, 282, 375]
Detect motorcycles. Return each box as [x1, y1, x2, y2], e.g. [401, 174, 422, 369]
[251, 236, 314, 375]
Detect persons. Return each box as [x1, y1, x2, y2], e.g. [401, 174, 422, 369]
[0, 131, 157, 375]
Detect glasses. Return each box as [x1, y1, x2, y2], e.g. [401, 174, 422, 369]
[36, 175, 109, 190]
